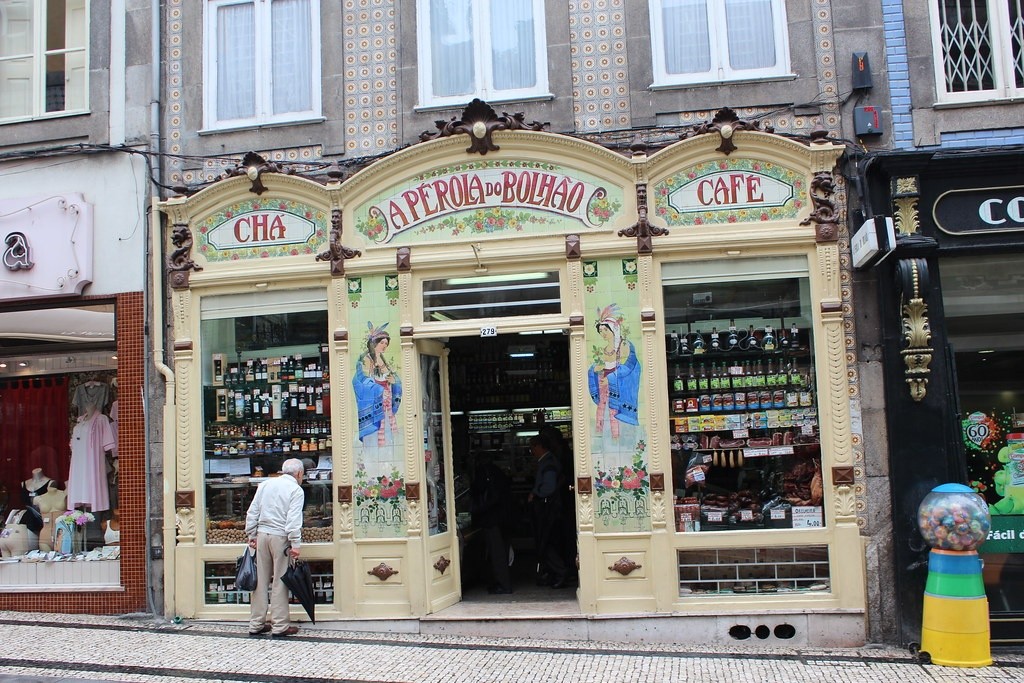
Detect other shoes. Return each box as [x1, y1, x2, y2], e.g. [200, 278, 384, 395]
[249, 625, 272, 636]
[536, 578, 552, 586]
[271, 627, 298, 637]
[553, 576, 569, 589]
[489, 587, 512, 594]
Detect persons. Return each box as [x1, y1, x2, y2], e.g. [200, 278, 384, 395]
[0, 523, 29, 557]
[21, 468, 67, 554]
[66, 404, 116, 512]
[101, 509, 120, 547]
[245, 458, 305, 638]
[525, 428, 573, 589]
[302, 458, 330, 502]
[470, 450, 525, 594]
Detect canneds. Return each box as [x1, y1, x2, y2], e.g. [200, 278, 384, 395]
[213, 435, 333, 456]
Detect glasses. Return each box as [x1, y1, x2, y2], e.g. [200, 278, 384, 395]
[529, 446, 536, 453]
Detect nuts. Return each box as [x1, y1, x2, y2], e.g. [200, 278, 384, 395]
[205, 526, 332, 544]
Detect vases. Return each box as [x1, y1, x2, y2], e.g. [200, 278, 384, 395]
[72, 531, 83, 555]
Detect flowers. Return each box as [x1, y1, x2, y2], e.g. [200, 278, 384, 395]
[56, 510, 95, 530]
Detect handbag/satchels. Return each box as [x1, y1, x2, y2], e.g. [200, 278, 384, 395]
[539, 465, 569, 503]
[237, 545, 258, 591]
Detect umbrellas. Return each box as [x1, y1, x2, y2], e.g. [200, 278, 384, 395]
[280, 545, 315, 625]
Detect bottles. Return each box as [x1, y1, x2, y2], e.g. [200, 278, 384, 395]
[206, 354, 332, 456]
[1007, 433, 1024, 487]
[672, 358, 812, 395]
[475, 349, 570, 404]
[208, 568, 334, 603]
[670, 318, 799, 353]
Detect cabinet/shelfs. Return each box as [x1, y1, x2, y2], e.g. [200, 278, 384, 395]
[468, 406, 572, 486]
[670, 384, 820, 451]
